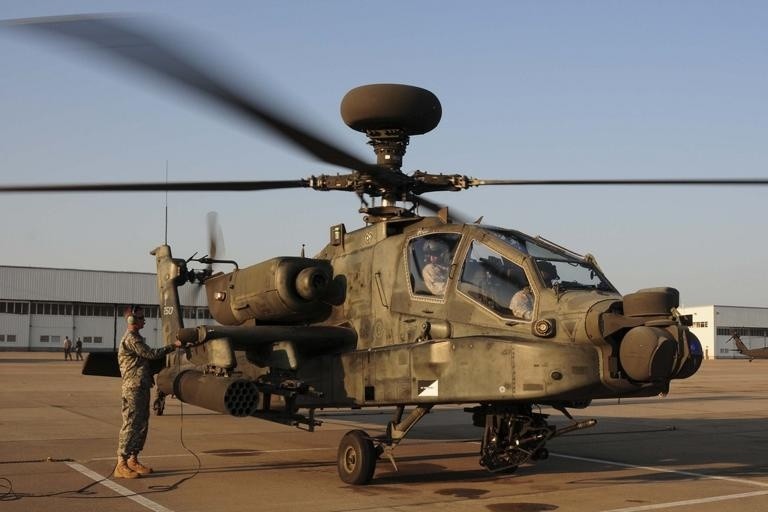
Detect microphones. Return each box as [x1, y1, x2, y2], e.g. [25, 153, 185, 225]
[144, 323, 145, 325]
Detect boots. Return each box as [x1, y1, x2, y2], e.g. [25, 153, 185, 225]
[114, 456, 143, 479]
[127, 455, 154, 474]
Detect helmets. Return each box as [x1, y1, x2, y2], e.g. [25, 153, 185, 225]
[423, 240, 445, 261]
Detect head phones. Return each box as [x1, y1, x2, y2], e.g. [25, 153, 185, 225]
[126, 305, 137, 326]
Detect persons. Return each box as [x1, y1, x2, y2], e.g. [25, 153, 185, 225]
[113, 305, 185, 479]
[422, 239, 451, 296]
[64, 336, 72, 361]
[75, 337, 83, 360]
[509, 262, 557, 321]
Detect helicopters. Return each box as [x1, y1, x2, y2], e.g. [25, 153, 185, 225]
[725, 332, 768, 363]
[0, 17, 767, 486]
